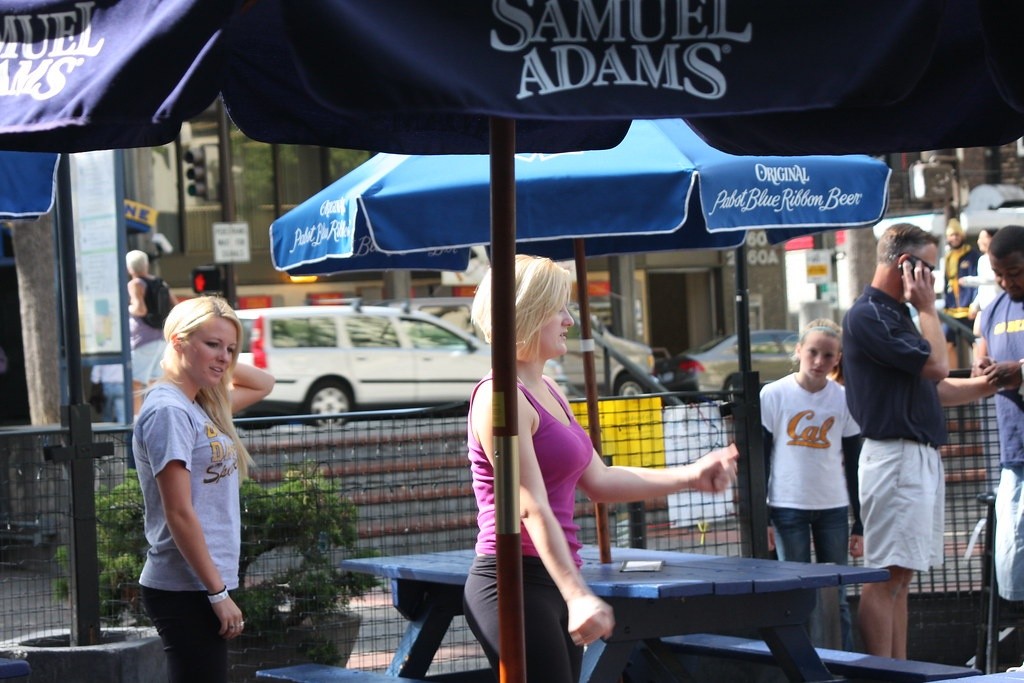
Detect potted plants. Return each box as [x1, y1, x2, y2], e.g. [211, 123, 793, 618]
[49, 456, 391, 683]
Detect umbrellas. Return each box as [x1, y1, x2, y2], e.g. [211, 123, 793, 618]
[269, 118, 894, 276]
[0, 0, 1024, 157]
[0, 151, 61, 217]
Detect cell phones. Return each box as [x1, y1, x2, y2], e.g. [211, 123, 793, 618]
[899, 254, 935, 280]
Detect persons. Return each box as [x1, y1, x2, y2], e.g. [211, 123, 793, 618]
[842, 223, 1022, 661]
[131, 296, 276, 683]
[759, 318, 864, 652]
[978, 224, 1024, 673]
[126, 250, 180, 416]
[462, 254, 740, 683]
[941, 217, 1002, 371]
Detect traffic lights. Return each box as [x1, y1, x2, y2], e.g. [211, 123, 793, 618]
[193, 267, 219, 295]
[184, 144, 206, 199]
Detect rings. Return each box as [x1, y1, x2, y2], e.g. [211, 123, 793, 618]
[230, 626, 233, 628]
[236, 625, 239, 627]
[240, 622, 244, 626]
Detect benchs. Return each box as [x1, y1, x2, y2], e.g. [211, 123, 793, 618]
[255, 662, 427, 683]
[660, 634, 984, 683]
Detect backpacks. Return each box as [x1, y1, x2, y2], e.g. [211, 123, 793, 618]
[134, 274, 171, 329]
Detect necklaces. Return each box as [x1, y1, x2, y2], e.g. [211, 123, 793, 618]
[192, 400, 194, 404]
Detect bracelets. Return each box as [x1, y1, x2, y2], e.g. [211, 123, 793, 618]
[207, 585, 229, 604]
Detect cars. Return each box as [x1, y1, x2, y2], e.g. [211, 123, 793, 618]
[381, 297, 654, 406]
[231, 306, 570, 427]
[674, 327, 801, 401]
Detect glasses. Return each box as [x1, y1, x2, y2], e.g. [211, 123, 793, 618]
[899, 252, 936, 273]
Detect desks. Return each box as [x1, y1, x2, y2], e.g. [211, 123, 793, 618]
[339, 543, 892, 683]
